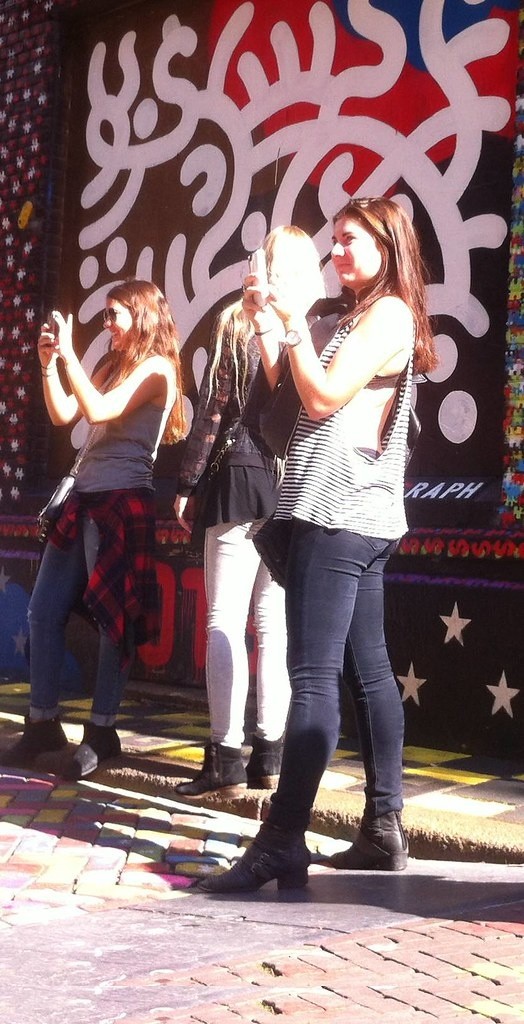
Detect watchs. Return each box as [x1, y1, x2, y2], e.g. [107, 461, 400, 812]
[285, 329, 312, 348]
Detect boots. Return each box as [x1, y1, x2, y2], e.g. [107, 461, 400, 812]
[244, 733, 284, 789]
[61, 720, 120, 780]
[0, 713, 66, 766]
[174, 743, 248, 799]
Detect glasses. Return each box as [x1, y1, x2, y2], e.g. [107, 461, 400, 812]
[103, 307, 131, 322]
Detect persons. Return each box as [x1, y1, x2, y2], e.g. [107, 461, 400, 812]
[198, 196, 441, 894]
[0, 279, 188, 780]
[173, 226, 328, 800]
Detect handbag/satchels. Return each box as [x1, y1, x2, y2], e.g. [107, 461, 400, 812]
[37, 475, 75, 546]
[186, 462, 220, 556]
[252, 509, 295, 589]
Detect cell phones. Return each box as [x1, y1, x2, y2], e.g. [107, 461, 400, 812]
[47, 310, 57, 346]
[248, 249, 268, 302]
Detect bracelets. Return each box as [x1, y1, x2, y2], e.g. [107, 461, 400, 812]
[254, 329, 272, 336]
[41, 365, 58, 376]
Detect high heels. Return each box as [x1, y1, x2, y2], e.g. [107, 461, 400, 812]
[197, 820, 310, 892]
[329, 810, 407, 871]
